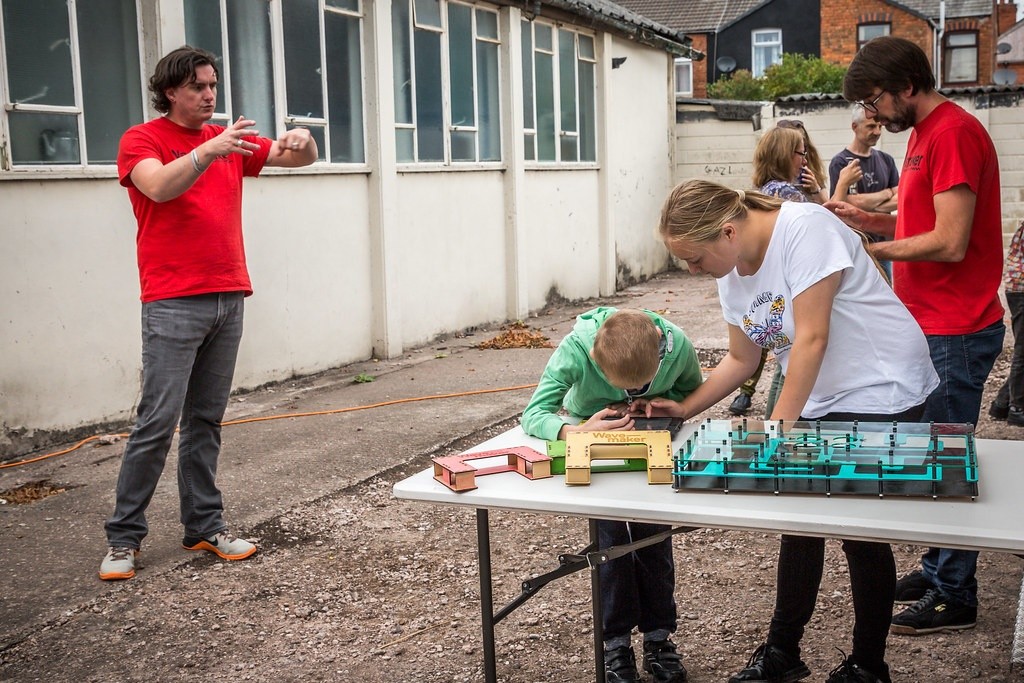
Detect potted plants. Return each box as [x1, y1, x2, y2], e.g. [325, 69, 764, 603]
[708, 69, 774, 122]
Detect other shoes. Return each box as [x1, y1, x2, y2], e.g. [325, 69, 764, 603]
[989, 402, 1024, 425]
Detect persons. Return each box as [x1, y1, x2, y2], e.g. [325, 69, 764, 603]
[987, 219, 1024, 427]
[829, 101, 899, 285]
[631, 177, 941, 683]
[823, 36, 1005, 635]
[521, 304, 703, 683]
[754, 127, 826, 206]
[777, 119, 825, 188]
[100, 49, 319, 580]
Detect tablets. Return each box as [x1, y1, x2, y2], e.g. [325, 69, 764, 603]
[597, 416, 684, 441]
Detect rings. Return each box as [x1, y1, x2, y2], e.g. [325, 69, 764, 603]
[237, 140, 243, 146]
[292, 143, 299, 146]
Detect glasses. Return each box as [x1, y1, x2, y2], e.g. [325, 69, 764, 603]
[792, 149, 807, 162]
[857, 88, 887, 115]
[778, 120, 803, 129]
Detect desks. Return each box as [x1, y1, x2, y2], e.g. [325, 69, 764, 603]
[393, 414, 1024, 683]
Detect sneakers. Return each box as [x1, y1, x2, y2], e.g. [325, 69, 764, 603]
[99, 545, 138, 580]
[889, 590, 977, 634]
[893, 570, 936, 605]
[605, 645, 639, 683]
[181, 532, 256, 561]
[642, 640, 687, 682]
[729, 642, 812, 683]
[824, 646, 891, 683]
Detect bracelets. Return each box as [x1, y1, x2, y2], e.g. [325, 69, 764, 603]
[890, 188, 894, 196]
[189, 149, 207, 173]
[811, 188, 821, 194]
